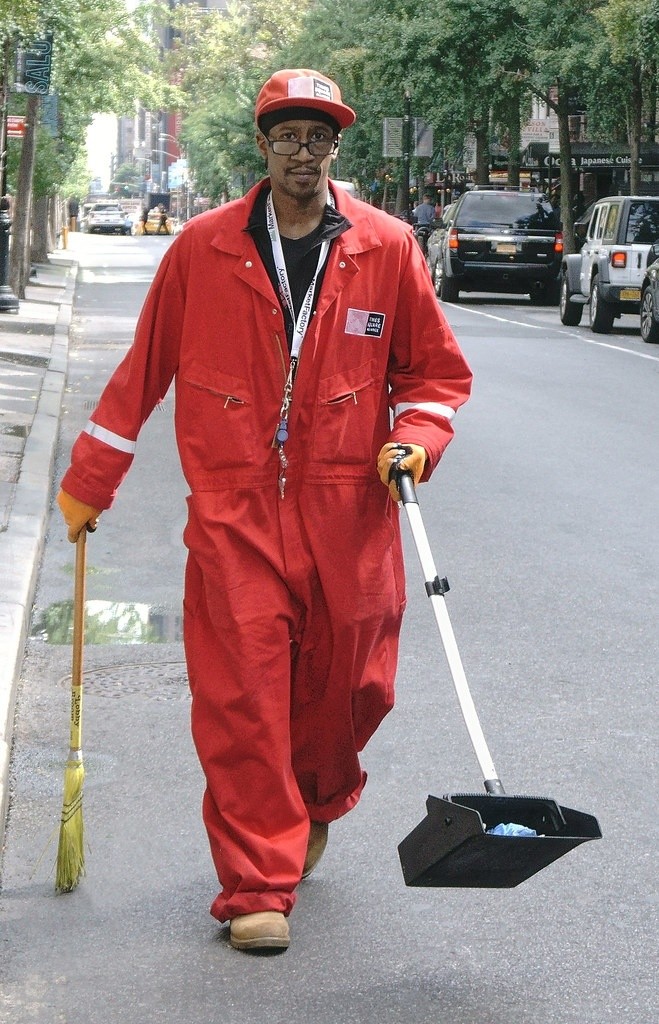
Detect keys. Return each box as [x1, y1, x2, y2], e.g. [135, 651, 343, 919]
[278, 478, 286, 500]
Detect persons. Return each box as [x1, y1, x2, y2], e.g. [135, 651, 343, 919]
[141, 206, 148, 235]
[154, 203, 170, 235]
[55, 68, 473, 951]
[409, 193, 441, 224]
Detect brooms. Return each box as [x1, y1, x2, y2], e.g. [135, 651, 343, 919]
[27, 521, 86, 896]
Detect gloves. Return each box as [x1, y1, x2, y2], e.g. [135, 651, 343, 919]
[376, 442, 427, 502]
[57, 488, 103, 543]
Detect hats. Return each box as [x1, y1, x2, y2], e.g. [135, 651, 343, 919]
[255, 68, 356, 129]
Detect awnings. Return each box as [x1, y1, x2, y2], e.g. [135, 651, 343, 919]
[492, 142, 659, 169]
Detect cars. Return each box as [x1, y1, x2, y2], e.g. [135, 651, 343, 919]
[87, 202, 127, 235]
[641, 237, 659, 344]
[415, 191, 562, 303]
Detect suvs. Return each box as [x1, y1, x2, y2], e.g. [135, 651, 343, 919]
[558, 196, 659, 335]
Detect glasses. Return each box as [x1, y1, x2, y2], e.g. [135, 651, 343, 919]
[259, 129, 339, 157]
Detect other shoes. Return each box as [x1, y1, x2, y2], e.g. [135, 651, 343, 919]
[228, 912, 291, 949]
[300, 823, 330, 880]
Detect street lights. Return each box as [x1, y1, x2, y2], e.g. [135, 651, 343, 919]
[134, 132, 190, 220]
